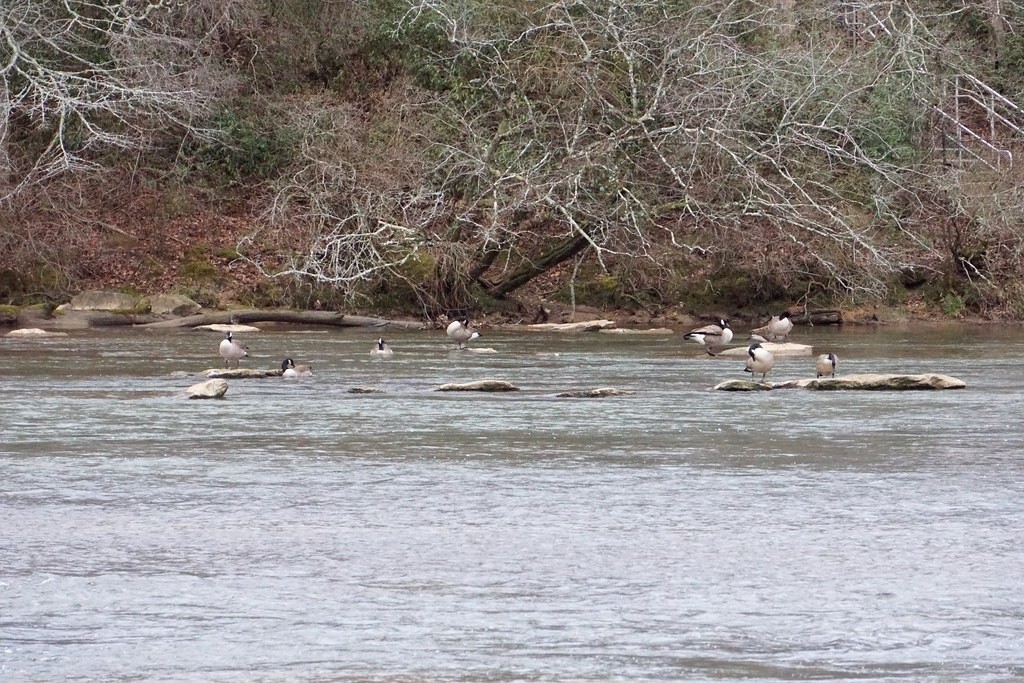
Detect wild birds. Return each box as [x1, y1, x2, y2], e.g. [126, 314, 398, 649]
[219, 330, 249, 369]
[744, 343, 774, 383]
[371, 337, 393, 354]
[446, 315, 483, 349]
[682, 318, 733, 356]
[748, 311, 794, 343]
[282, 358, 312, 377]
[815, 353, 840, 378]
